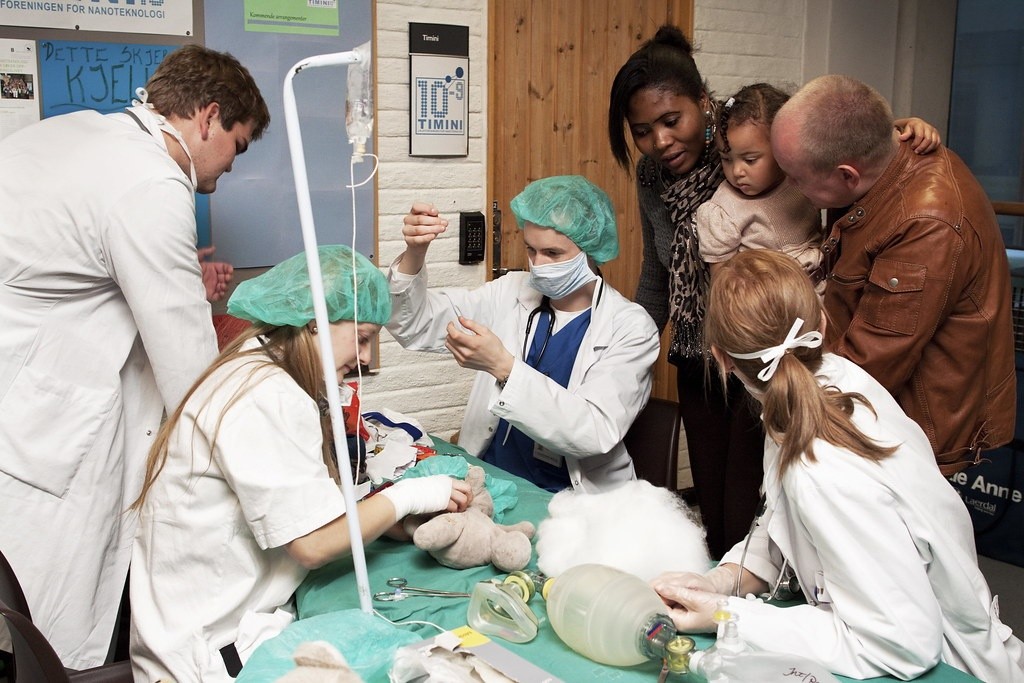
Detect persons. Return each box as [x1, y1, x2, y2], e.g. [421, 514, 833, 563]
[128, 242, 474, 683]
[650, 246, 1024, 683]
[621, 42, 765, 561]
[771, 74, 1017, 477]
[691, 80, 829, 323]
[1, 43, 271, 683]
[384, 175, 661, 496]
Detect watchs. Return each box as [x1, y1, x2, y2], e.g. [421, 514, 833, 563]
[494, 375, 509, 391]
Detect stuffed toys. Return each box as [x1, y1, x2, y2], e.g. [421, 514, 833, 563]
[402, 464, 536, 571]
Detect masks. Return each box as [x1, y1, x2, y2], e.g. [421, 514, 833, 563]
[526, 250, 597, 301]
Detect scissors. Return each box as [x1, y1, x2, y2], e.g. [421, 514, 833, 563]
[373, 577, 472, 602]
